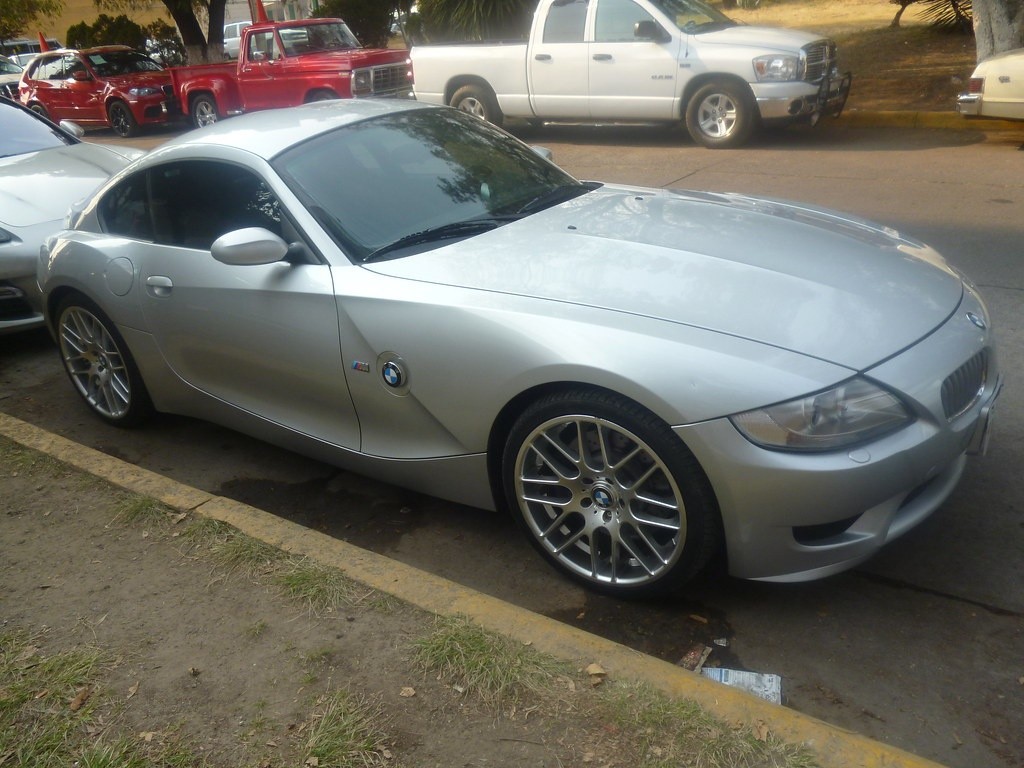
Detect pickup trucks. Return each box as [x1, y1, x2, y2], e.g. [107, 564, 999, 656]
[222, 21, 309, 60]
[162, 18, 414, 130]
[407, 0, 852, 149]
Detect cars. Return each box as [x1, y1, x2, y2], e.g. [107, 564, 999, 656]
[18, 45, 183, 138]
[956, 47, 1024, 122]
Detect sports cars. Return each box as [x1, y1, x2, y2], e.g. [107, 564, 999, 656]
[0, 95, 150, 334]
[37, 99, 1002, 608]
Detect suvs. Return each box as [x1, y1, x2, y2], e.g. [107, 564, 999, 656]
[0, 38, 64, 102]
